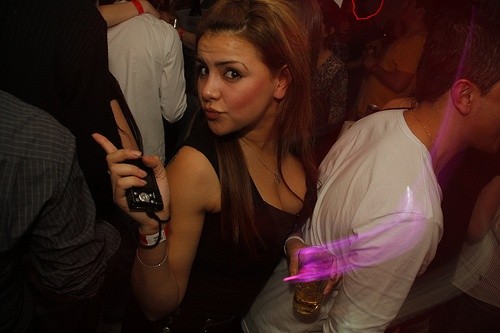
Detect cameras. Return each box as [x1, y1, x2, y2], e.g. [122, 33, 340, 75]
[119, 156, 164, 213]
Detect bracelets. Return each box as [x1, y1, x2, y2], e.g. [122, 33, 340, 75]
[172, 17, 177, 28]
[138, 224, 169, 246]
[136, 246, 169, 268]
[131, 0, 144, 15]
[284, 236, 304, 257]
[178, 27, 185, 39]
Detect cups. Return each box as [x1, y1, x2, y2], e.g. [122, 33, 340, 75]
[293, 245, 335, 320]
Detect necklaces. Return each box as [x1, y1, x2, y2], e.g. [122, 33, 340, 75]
[409, 107, 439, 159]
[240, 137, 281, 185]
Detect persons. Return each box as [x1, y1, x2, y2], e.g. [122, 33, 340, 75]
[0, 0, 500, 333]
